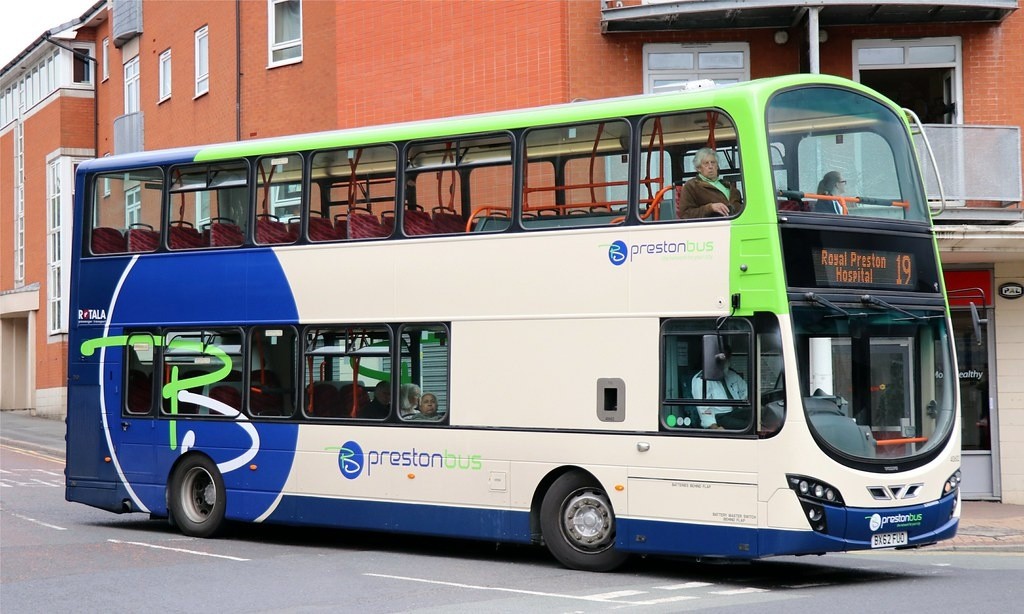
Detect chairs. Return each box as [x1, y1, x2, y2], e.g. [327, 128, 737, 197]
[125, 369, 372, 421]
[679, 363, 749, 428]
[79, 179, 807, 253]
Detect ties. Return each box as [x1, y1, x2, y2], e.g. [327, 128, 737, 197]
[721, 377, 738, 408]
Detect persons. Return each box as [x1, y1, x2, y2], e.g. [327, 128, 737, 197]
[400, 383, 421, 420]
[691, 346, 748, 430]
[816, 171, 849, 216]
[411, 393, 443, 421]
[679, 147, 742, 219]
[306, 380, 391, 419]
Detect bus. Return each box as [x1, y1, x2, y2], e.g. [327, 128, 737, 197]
[65, 72, 965, 572]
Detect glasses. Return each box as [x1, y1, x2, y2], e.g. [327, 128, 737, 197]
[838, 179, 847, 185]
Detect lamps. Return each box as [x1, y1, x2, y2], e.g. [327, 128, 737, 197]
[819, 29, 828, 43]
[775, 30, 787, 46]
